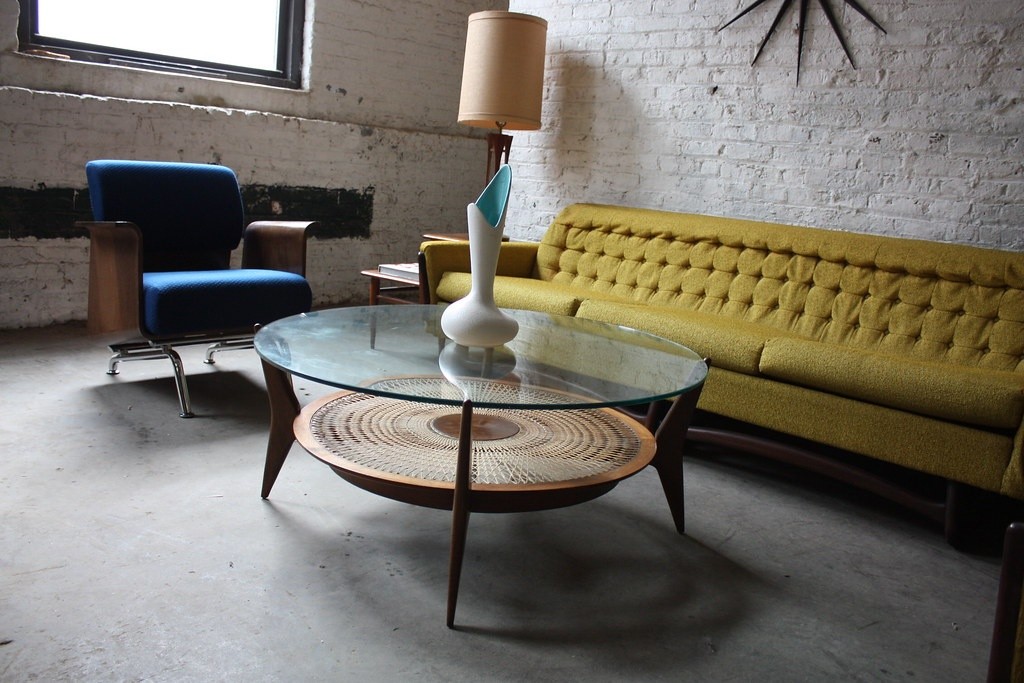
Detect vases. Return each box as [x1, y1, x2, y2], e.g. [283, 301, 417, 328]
[439, 164, 519, 346]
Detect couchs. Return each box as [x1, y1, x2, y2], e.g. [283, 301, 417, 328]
[417, 203, 1024, 558]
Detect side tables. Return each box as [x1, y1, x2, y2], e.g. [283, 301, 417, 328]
[360, 233, 524, 349]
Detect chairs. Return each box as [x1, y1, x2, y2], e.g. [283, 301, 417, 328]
[74, 159, 322, 421]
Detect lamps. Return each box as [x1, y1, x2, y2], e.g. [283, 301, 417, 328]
[420, 11, 553, 243]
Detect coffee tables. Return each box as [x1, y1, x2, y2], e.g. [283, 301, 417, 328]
[255, 304, 712, 628]
